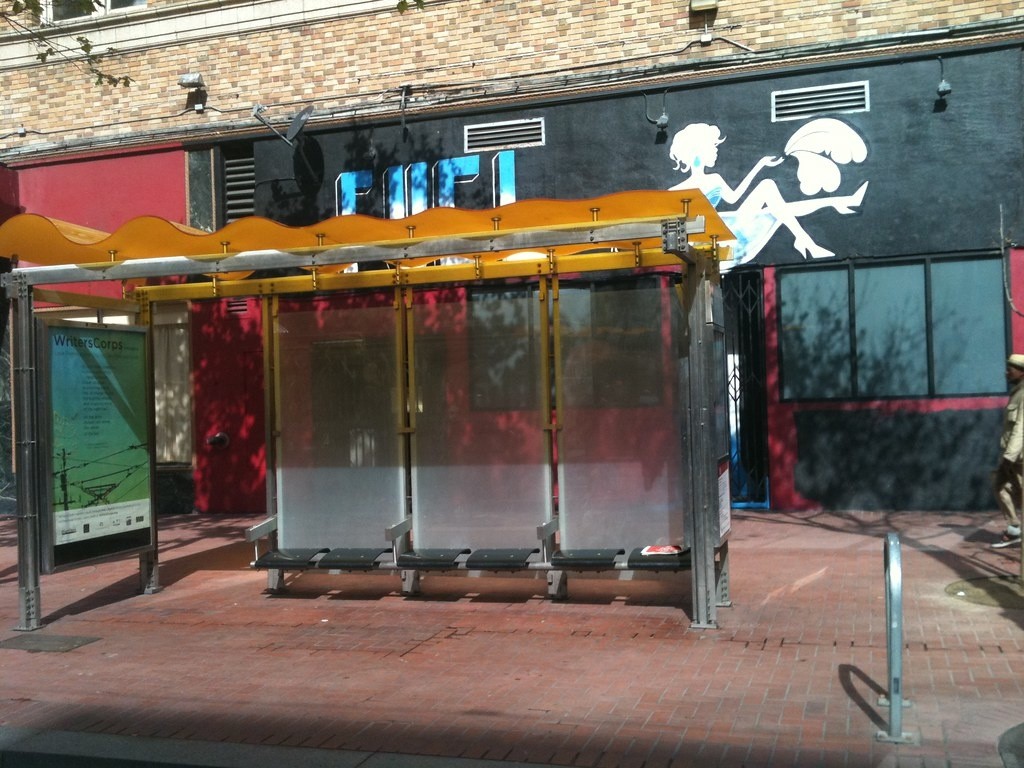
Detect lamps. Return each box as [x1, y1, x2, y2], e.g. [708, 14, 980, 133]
[936, 57, 952, 93]
[657, 91, 669, 126]
[689, 0, 717, 11]
[177, 73, 204, 87]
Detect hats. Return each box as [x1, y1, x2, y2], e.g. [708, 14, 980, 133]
[1005, 354, 1024, 370]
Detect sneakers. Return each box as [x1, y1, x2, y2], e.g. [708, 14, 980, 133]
[990, 530, 1022, 548]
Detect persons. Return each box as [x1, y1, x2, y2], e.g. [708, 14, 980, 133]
[991, 354, 1024, 548]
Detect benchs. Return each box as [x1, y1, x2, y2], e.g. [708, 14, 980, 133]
[245, 513, 691, 572]
